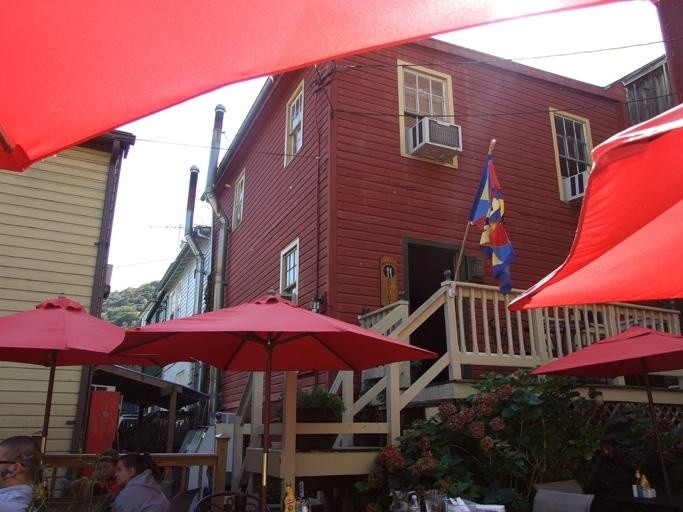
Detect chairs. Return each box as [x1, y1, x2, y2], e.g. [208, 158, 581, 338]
[166, 479, 596, 512]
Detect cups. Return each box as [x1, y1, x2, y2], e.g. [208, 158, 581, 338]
[425, 490, 477, 512]
[632, 486, 637, 497]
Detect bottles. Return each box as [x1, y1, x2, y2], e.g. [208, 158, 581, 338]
[635, 469, 648, 498]
[388, 489, 421, 512]
[280, 481, 312, 512]
[42, 476, 49, 499]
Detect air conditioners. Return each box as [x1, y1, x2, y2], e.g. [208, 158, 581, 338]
[409, 116, 463, 162]
[561, 171, 590, 203]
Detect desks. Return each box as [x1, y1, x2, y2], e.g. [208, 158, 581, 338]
[607, 494, 683, 512]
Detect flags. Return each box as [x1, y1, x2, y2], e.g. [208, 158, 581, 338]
[470, 154, 517, 297]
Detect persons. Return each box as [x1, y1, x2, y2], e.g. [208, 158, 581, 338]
[0, 436, 43, 512]
[109, 450, 173, 512]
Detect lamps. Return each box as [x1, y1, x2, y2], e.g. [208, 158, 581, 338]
[309, 294, 325, 313]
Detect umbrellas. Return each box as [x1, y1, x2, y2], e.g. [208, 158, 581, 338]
[1, 294, 162, 455]
[528, 328, 682, 505]
[119, 290, 438, 512]
[505, 97, 683, 313]
